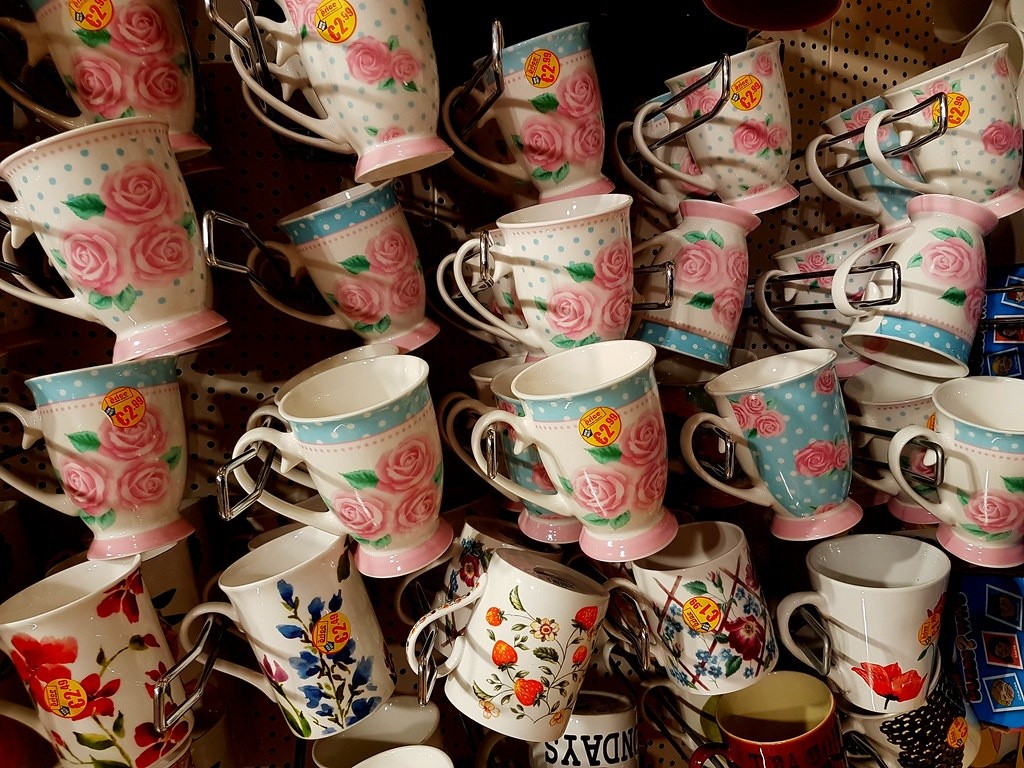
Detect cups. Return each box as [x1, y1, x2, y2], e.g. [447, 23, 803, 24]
[0, 0, 1024, 768]
